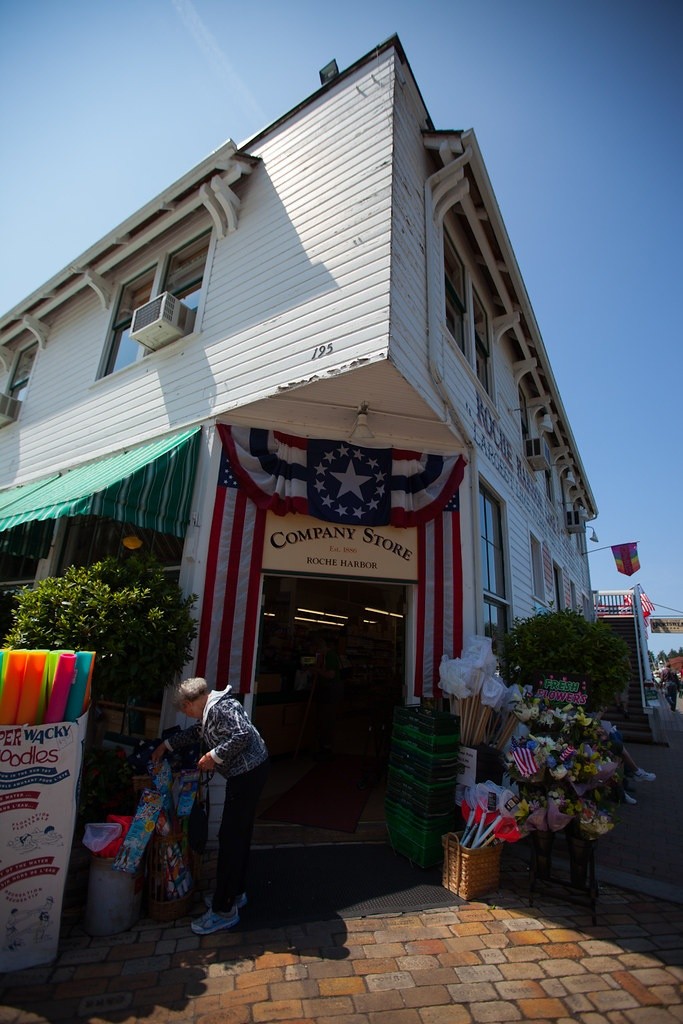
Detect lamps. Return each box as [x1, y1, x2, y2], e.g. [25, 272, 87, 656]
[319, 58, 339, 85]
[348, 401, 376, 439]
[551, 464, 576, 486]
[585, 526, 599, 542]
[123, 534, 143, 550]
[510, 404, 553, 433]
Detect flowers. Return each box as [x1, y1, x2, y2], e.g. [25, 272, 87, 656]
[503, 675, 626, 840]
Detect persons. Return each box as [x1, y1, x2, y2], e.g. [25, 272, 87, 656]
[151, 675, 267, 936]
[613, 654, 632, 721]
[603, 724, 638, 793]
[658, 662, 681, 712]
[586, 704, 657, 804]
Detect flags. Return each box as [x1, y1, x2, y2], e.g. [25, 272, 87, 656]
[413, 482, 464, 701]
[197, 442, 267, 695]
[623, 583, 655, 613]
[510, 735, 538, 776]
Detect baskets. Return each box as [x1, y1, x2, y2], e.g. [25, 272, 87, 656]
[442, 832, 503, 900]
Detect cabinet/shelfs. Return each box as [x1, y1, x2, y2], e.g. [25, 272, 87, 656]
[529, 833, 598, 926]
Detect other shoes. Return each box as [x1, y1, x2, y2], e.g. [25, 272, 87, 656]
[624, 791, 637, 804]
[633, 769, 656, 782]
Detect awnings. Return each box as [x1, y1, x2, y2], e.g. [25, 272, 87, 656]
[0, 424, 205, 545]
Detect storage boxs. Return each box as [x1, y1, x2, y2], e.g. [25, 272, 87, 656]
[458, 743, 505, 787]
[385, 704, 461, 868]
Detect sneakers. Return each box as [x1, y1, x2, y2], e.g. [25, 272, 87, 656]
[205, 891, 247, 908]
[191, 906, 239, 934]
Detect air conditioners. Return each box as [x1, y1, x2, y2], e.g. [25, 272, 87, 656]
[565, 510, 586, 534]
[0, 392, 21, 426]
[129, 291, 194, 353]
[525, 437, 552, 471]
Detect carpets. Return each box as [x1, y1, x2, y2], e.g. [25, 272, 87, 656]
[257, 753, 373, 834]
[228, 844, 469, 932]
